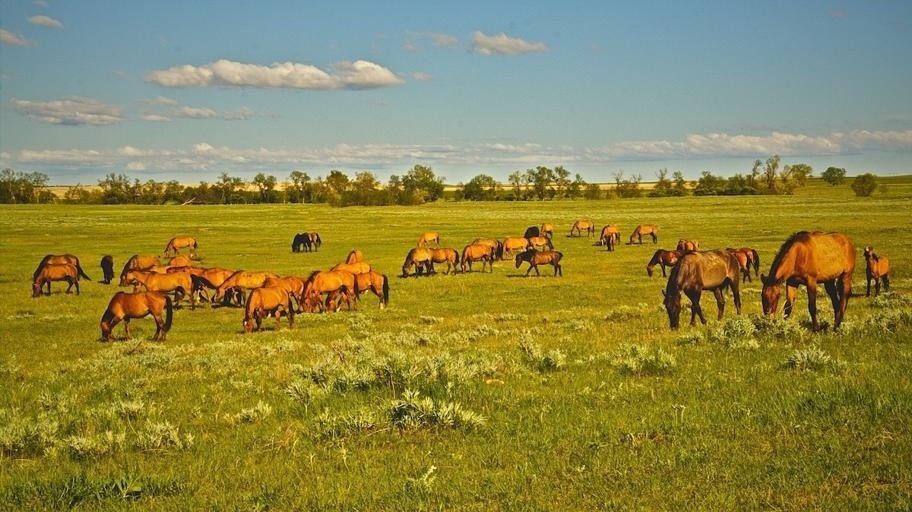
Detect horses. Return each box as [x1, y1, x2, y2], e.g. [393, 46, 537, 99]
[598, 223, 621, 251]
[163, 236, 200, 257]
[33, 254, 91, 283]
[402, 221, 564, 278]
[645, 238, 760, 331]
[32, 263, 81, 297]
[99, 250, 389, 344]
[291, 232, 322, 252]
[630, 225, 658, 245]
[863, 245, 891, 298]
[570, 220, 595, 238]
[761, 230, 857, 333]
